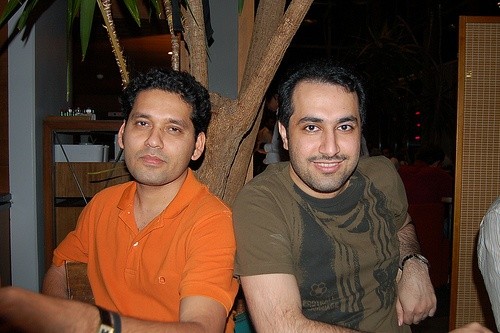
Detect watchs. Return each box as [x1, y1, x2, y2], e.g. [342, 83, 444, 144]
[95, 305, 114, 333]
[401, 253, 428, 269]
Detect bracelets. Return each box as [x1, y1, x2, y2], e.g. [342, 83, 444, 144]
[113, 312, 122, 333]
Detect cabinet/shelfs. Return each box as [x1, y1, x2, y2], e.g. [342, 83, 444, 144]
[45, 116, 133, 275]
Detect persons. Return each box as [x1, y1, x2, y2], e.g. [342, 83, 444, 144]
[254, 90, 288, 174]
[381, 144, 456, 245]
[0, 69, 237, 333]
[232, 67, 493, 333]
